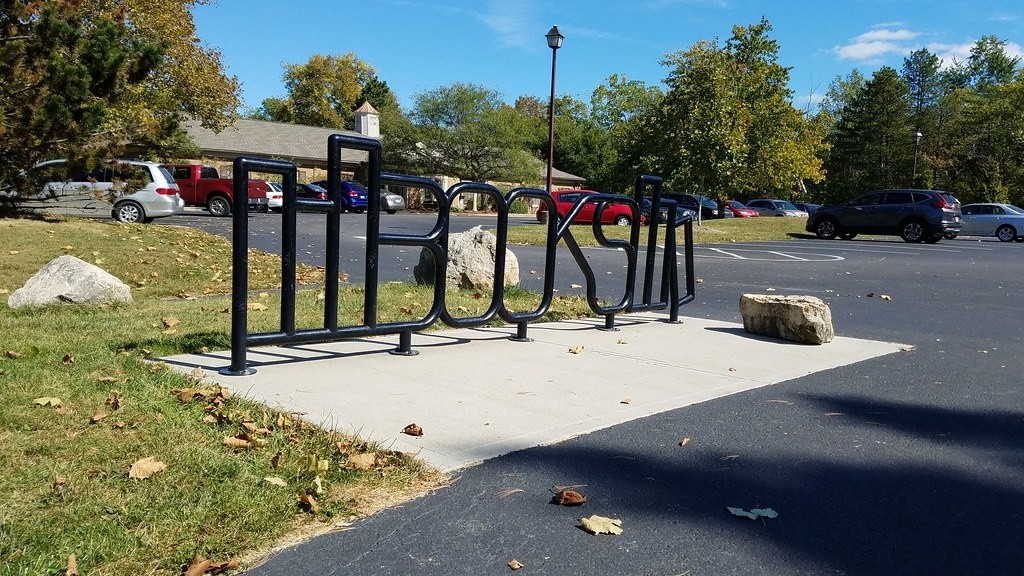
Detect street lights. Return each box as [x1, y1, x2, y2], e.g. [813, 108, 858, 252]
[912, 132, 922, 187]
[543, 24, 566, 194]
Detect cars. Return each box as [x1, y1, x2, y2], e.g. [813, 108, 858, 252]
[311, 179, 370, 213]
[260, 181, 287, 213]
[946, 201, 1024, 243]
[0, 159, 185, 227]
[362, 186, 405, 215]
[535, 190, 645, 229]
[291, 182, 337, 214]
[628, 188, 822, 225]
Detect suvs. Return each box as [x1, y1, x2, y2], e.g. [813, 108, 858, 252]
[806, 188, 962, 245]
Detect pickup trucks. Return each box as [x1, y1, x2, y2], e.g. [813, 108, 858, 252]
[154, 164, 267, 217]
